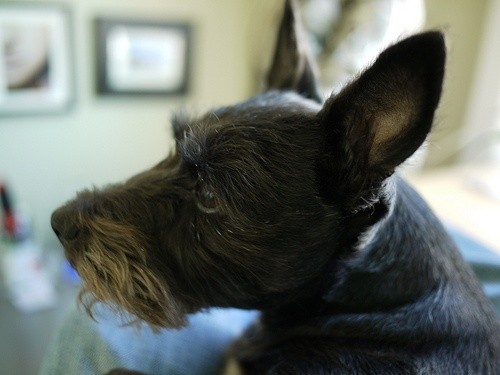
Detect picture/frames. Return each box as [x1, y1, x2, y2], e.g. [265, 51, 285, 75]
[95, 18, 195, 97]
[0, 0, 80, 118]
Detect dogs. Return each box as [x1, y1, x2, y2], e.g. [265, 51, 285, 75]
[50, 0, 500, 375]
